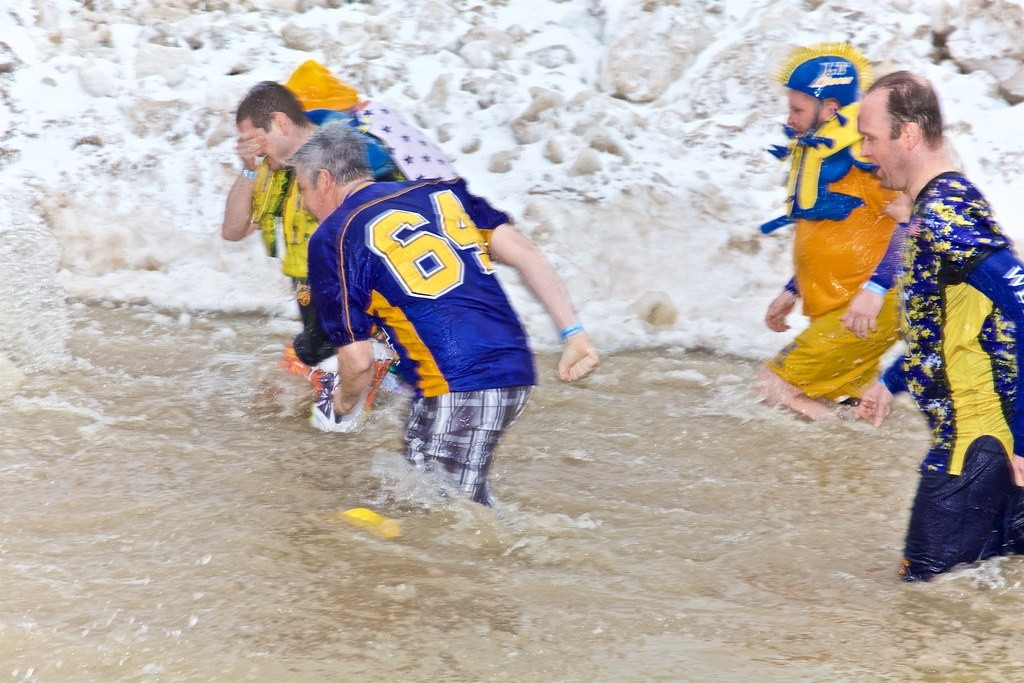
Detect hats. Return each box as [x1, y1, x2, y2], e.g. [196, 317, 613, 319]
[283, 61, 359, 114]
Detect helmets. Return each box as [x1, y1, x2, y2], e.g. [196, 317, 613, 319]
[773, 42, 874, 109]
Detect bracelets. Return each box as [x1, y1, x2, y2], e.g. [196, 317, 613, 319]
[241, 166, 259, 182]
[561, 321, 584, 342]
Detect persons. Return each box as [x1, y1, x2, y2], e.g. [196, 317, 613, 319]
[750, 62, 923, 435]
[854, 71, 1024, 581]
[219, 81, 412, 402]
[289, 61, 460, 193]
[287, 122, 599, 509]
